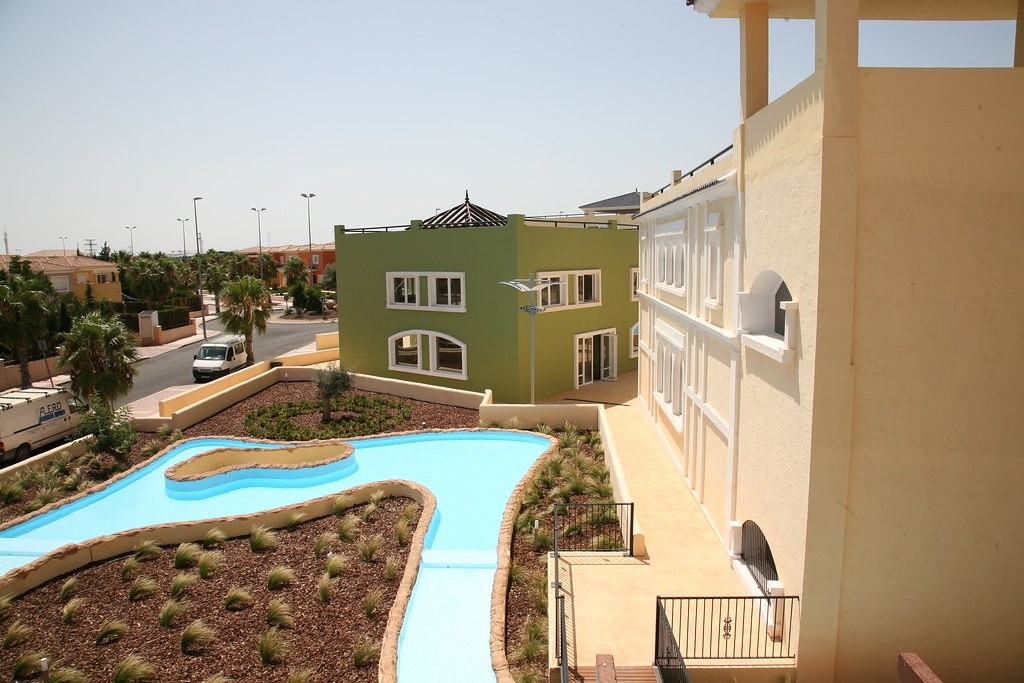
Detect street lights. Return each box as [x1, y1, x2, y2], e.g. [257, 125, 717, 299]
[300, 192, 315, 291]
[126, 226, 137, 255]
[177, 219, 190, 256]
[250, 207, 266, 286]
[59, 236, 67, 256]
[193, 197, 207, 341]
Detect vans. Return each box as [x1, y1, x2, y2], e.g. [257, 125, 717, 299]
[0, 376, 98, 464]
[192, 334, 249, 382]
